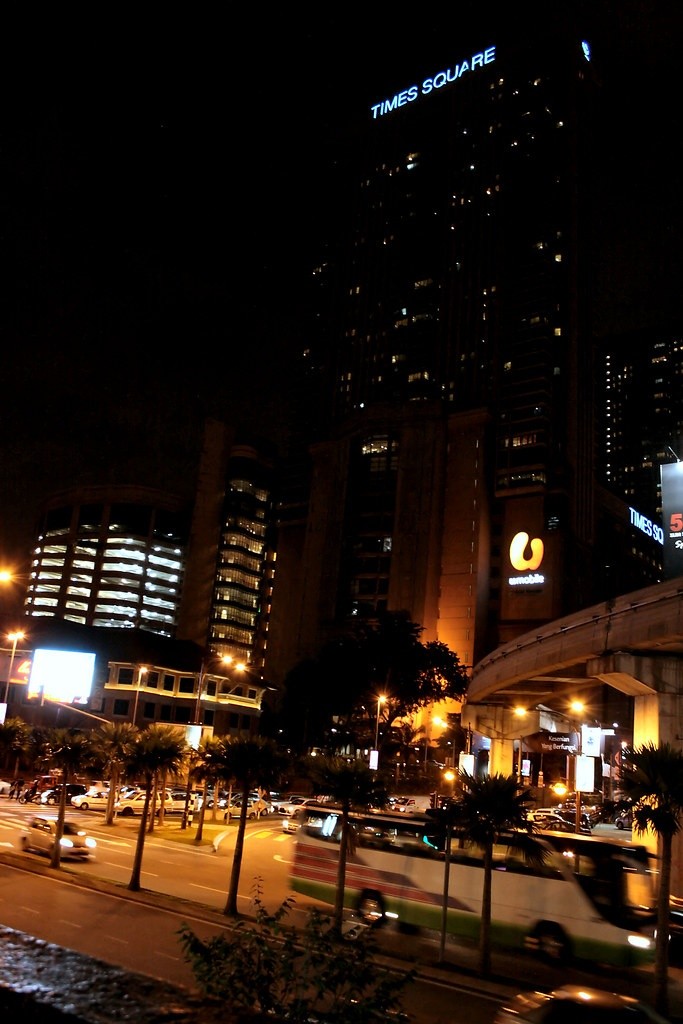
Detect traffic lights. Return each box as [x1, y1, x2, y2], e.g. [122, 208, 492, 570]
[35, 686, 45, 707]
[69, 697, 81, 710]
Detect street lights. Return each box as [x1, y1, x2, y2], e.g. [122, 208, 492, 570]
[134, 665, 147, 727]
[194, 651, 246, 723]
[514, 707, 582, 833]
[2, 631, 25, 702]
[434, 717, 455, 790]
[375, 694, 387, 751]
[554, 777, 581, 833]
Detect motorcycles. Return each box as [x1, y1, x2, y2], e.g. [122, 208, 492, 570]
[18, 790, 42, 804]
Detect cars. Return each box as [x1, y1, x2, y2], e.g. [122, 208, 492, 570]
[494, 990, 665, 1024]
[615, 813, 632, 829]
[533, 820, 592, 835]
[278, 796, 322, 816]
[527, 813, 569, 824]
[0, 776, 301, 819]
[536, 809, 562, 813]
[16, 814, 97, 860]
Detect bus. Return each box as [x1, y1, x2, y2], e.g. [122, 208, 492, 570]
[289, 804, 661, 970]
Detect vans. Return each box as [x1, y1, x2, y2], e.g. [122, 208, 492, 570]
[393, 797, 416, 813]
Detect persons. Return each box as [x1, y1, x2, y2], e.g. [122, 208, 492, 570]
[27, 780, 38, 801]
[0, 787, 4, 794]
[9, 778, 25, 802]
